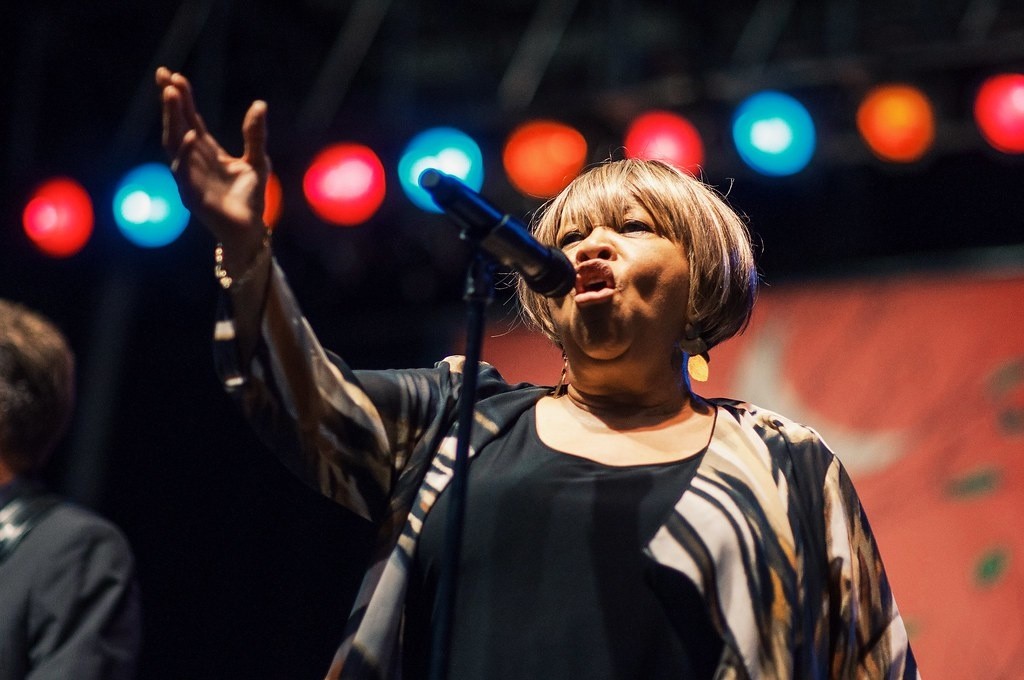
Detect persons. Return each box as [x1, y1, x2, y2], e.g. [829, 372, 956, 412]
[156, 63, 920, 680]
[0, 296, 146, 680]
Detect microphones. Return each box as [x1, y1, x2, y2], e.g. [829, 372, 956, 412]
[418, 168, 578, 300]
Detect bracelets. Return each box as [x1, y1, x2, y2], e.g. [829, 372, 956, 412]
[215, 221, 272, 290]
